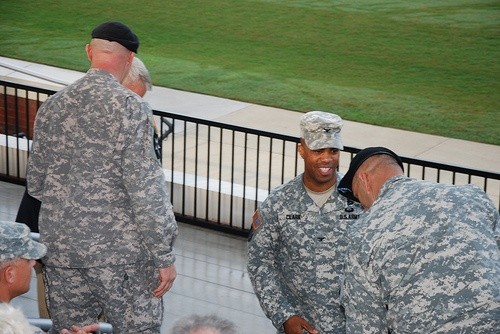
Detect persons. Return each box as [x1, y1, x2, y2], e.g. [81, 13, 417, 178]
[246, 111, 367, 334]
[336, 147, 500, 334]
[0, 220, 100, 334]
[13, 21, 178, 334]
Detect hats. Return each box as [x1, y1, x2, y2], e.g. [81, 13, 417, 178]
[0, 220, 48, 262]
[300, 110, 343, 150]
[337, 147, 404, 203]
[90, 21, 139, 54]
